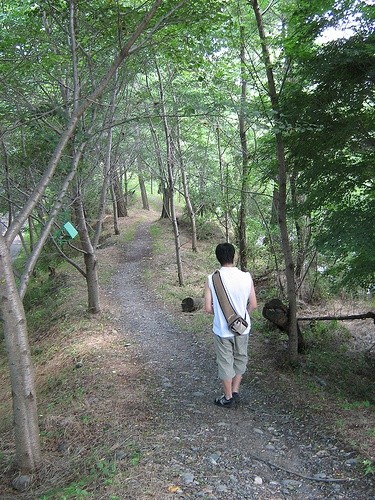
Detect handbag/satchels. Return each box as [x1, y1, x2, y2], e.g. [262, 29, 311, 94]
[228, 315, 248, 335]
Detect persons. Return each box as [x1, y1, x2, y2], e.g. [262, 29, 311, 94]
[204, 243, 258, 409]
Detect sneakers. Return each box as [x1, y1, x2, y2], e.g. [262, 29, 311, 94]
[232, 392, 241, 403]
[214, 395, 233, 408]
